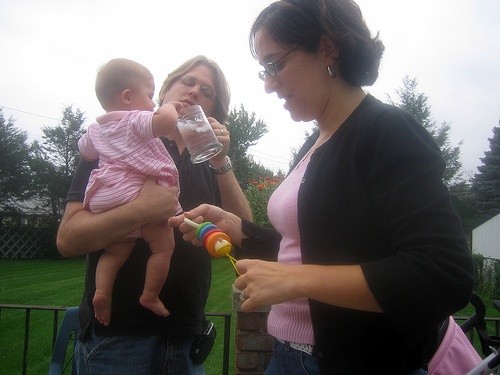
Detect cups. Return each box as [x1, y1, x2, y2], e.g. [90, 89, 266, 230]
[176, 104, 224, 165]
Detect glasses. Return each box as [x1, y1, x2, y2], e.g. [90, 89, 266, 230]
[180, 74, 217, 99]
[259, 51, 291, 81]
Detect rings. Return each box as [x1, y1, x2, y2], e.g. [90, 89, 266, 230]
[240, 290, 248, 301]
[220, 128, 223, 135]
[232, 284, 241, 294]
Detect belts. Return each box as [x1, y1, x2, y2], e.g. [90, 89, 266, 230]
[277, 339, 313, 356]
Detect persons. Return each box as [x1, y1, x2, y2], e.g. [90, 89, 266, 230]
[167, 0, 474, 375]
[77, 59, 183, 324]
[58, 54, 252, 375]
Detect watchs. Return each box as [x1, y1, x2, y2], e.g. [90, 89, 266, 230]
[209, 155, 232, 175]
